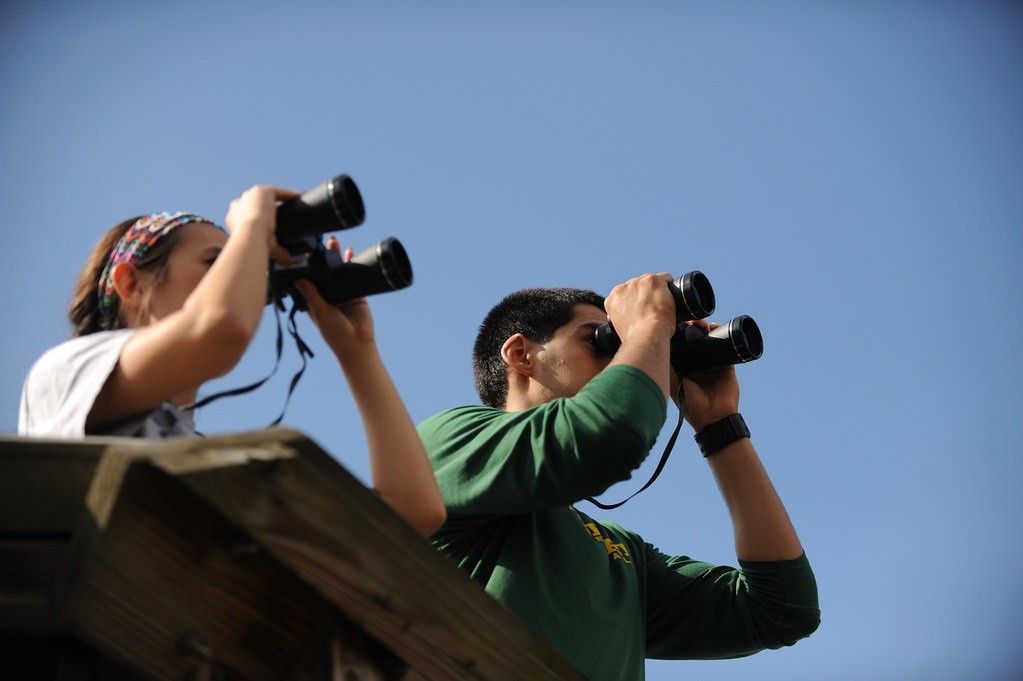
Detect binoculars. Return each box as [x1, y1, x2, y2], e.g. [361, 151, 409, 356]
[593, 269, 766, 381]
[263, 173, 414, 312]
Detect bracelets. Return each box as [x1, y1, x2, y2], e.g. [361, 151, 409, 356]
[694, 412, 752, 457]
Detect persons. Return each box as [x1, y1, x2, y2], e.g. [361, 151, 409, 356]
[413, 270, 821, 681]
[18, 184, 447, 536]
